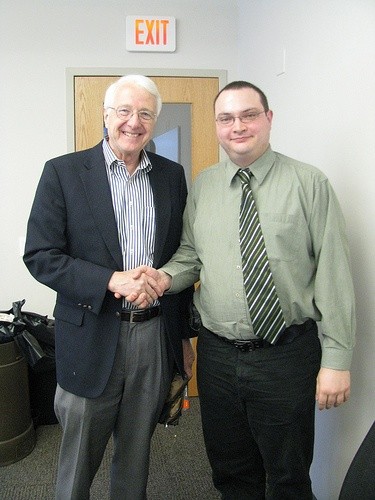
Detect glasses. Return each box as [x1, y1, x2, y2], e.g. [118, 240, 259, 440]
[216, 111, 266, 127]
[108, 106, 158, 120]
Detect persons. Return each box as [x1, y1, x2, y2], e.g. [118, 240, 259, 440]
[23, 74, 200, 500]
[114, 80, 358, 499]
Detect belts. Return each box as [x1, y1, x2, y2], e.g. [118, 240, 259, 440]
[120, 305, 160, 322]
[208, 329, 272, 352]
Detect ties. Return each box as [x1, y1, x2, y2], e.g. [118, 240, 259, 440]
[238, 169, 286, 344]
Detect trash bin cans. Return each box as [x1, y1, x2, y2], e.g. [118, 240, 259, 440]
[0, 321, 40, 466]
[14, 317, 61, 427]
